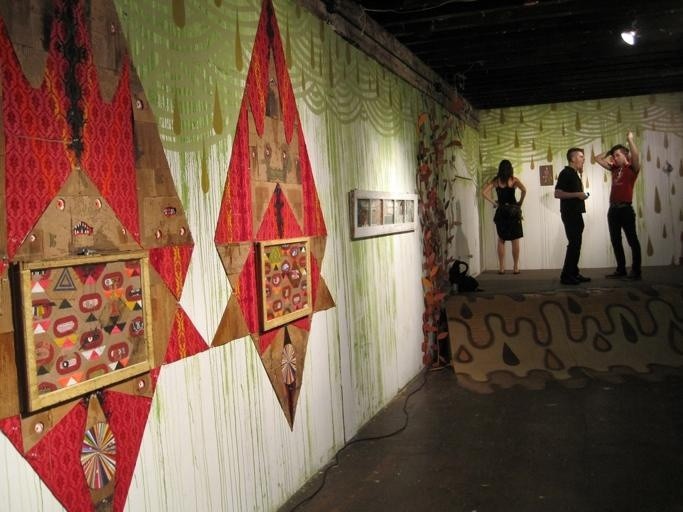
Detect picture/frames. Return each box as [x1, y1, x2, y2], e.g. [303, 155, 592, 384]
[350, 187, 418, 241]
[17, 249, 156, 412]
[257, 236, 315, 331]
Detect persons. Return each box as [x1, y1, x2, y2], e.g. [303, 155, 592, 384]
[542, 166, 551, 185]
[594, 128, 643, 280]
[482, 160, 527, 273]
[555, 146, 591, 286]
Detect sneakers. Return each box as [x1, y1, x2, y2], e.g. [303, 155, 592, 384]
[605, 270, 627, 279]
[560, 273, 591, 285]
[620, 270, 641, 281]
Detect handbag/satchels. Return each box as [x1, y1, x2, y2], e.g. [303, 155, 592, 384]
[502, 203, 523, 222]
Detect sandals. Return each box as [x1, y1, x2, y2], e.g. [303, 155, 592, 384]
[498, 269, 520, 274]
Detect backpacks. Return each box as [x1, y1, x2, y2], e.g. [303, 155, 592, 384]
[448, 260, 483, 292]
